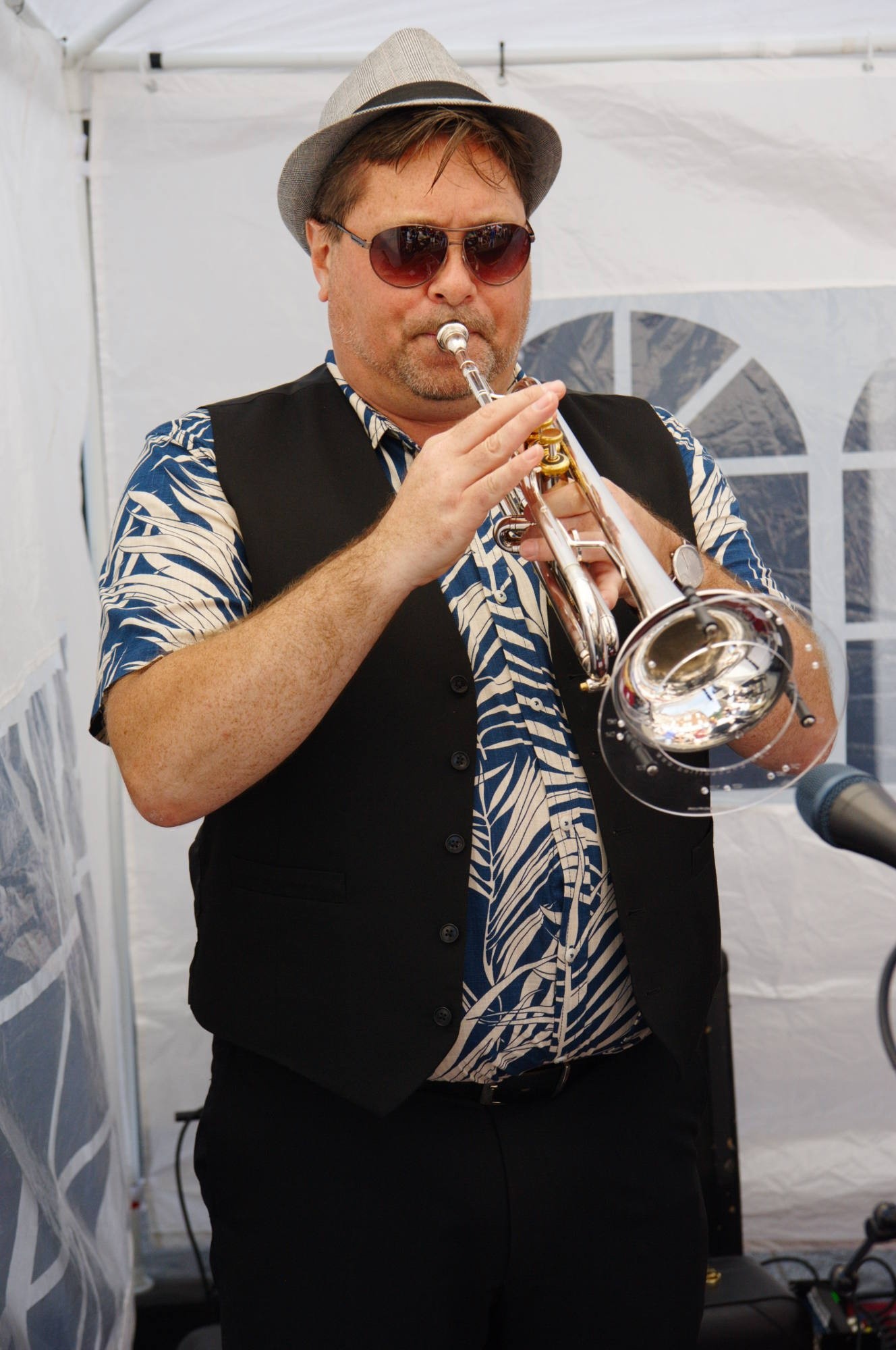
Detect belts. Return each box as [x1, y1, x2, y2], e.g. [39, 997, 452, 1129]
[415, 1052, 650, 1120]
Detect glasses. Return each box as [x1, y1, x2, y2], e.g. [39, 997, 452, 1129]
[321, 216, 535, 289]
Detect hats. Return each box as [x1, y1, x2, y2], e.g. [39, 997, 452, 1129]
[277, 28, 563, 258]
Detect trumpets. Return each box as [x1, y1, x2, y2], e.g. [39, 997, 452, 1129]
[434, 320, 794, 753]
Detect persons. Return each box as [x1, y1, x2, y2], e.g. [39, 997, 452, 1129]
[88, 24, 837, 1350]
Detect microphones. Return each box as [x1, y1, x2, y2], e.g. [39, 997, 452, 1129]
[795, 763, 896, 871]
